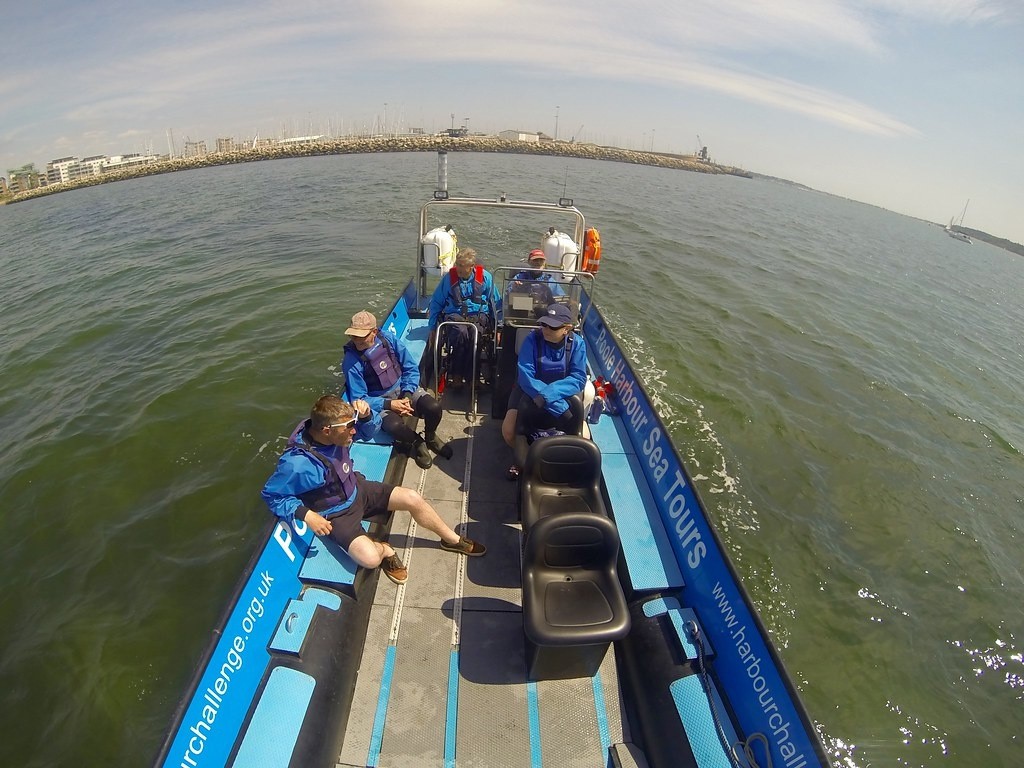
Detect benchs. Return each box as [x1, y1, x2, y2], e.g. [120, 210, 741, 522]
[230, 295, 438, 768]
[580, 331, 753, 768]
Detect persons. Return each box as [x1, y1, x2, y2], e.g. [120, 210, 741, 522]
[256, 393, 488, 586]
[427, 246, 501, 392]
[342, 311, 454, 470]
[503, 248, 569, 307]
[500, 303, 589, 482]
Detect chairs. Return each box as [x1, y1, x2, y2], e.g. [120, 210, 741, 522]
[521, 513, 633, 682]
[520, 434, 609, 538]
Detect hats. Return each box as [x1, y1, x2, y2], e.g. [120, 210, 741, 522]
[537, 304, 572, 327]
[529, 249, 546, 261]
[344, 310, 376, 337]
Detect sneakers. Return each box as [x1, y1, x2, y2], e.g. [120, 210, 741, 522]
[440, 536, 487, 557]
[380, 541, 409, 585]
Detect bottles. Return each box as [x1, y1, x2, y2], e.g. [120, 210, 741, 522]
[590, 396, 603, 424]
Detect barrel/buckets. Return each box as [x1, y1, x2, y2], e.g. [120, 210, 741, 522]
[423, 226, 456, 276]
[541, 230, 576, 282]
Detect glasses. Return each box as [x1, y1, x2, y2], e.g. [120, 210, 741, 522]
[327, 411, 359, 429]
[454, 261, 468, 268]
[541, 322, 563, 331]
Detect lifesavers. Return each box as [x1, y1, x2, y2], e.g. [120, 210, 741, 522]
[581, 228, 601, 278]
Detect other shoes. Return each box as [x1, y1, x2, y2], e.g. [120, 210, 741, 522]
[450, 376, 464, 390]
[425, 432, 453, 458]
[414, 442, 432, 470]
